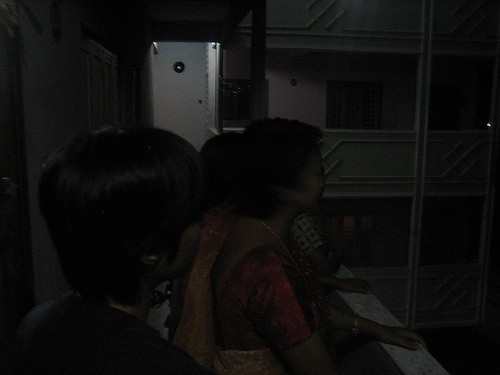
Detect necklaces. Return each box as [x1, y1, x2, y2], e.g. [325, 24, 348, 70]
[263, 221, 308, 283]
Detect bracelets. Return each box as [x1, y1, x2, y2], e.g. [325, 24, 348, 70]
[350, 314, 361, 336]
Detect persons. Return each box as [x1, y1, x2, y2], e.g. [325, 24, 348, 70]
[0, 124, 215, 375]
[209, 118, 429, 375]
[285, 167, 343, 277]
[166, 131, 372, 374]
